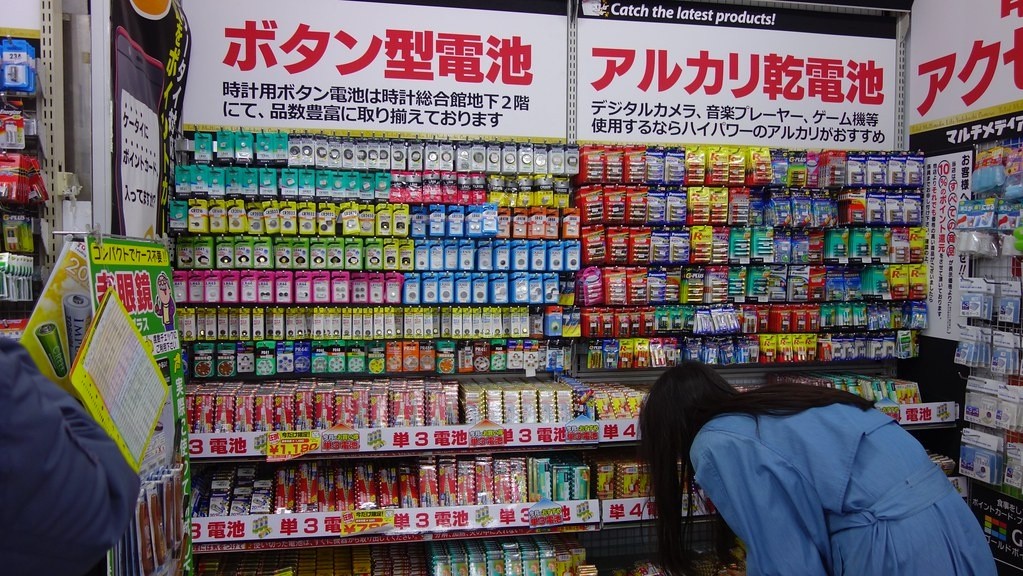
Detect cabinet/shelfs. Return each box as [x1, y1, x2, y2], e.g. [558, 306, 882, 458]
[0, 0, 1023, 576]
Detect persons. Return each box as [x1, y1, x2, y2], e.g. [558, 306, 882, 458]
[0, 335, 141, 576]
[639, 360, 1001, 576]
